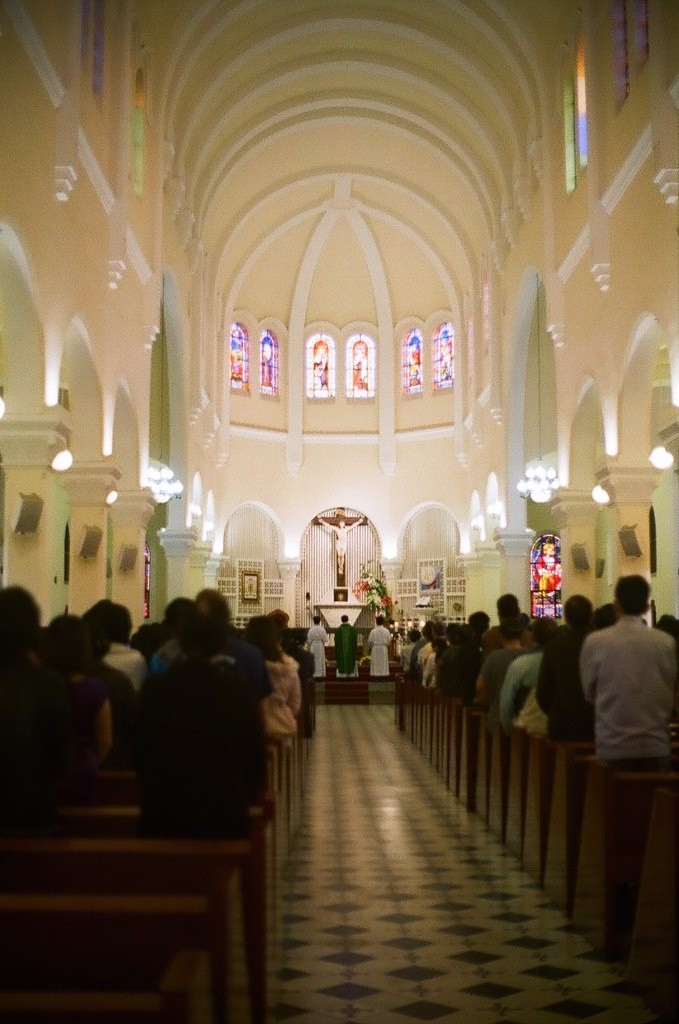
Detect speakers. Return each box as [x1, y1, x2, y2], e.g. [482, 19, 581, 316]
[11, 493, 44, 534]
[618, 524, 645, 557]
[595, 560, 605, 578]
[76, 524, 103, 558]
[568, 543, 592, 572]
[118, 545, 139, 570]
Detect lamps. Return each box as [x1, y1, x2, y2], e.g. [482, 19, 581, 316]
[483, 502, 503, 528]
[142, 279, 185, 503]
[516, 274, 561, 506]
[470, 515, 482, 541]
[190, 505, 200, 528]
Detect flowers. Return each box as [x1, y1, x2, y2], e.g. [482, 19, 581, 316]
[351, 560, 393, 618]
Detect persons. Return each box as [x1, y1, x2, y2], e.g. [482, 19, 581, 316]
[307, 616, 329, 681]
[538, 575, 679, 773]
[368, 615, 391, 682]
[0, 586, 315, 843]
[319, 518, 363, 574]
[334, 615, 358, 679]
[401, 594, 564, 741]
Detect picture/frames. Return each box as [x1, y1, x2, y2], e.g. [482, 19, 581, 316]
[242, 570, 260, 604]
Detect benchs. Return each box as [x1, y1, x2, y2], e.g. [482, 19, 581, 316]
[0, 675, 316, 1024]
[394, 672, 679, 1024]
